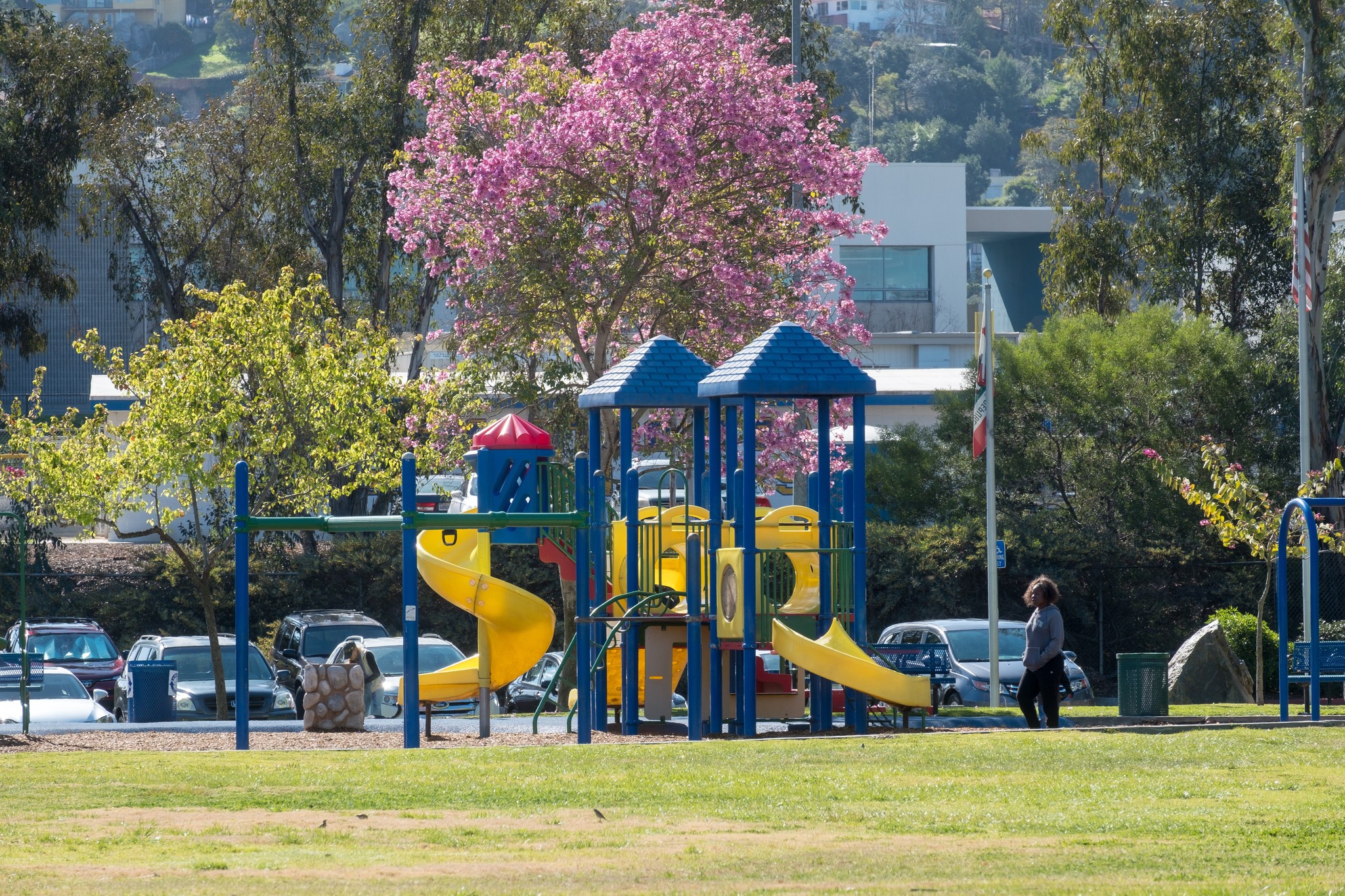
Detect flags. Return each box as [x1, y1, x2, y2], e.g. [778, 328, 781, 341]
[973, 302, 999, 460]
[1290, 159, 1314, 313]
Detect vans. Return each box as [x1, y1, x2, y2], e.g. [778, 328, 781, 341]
[867, 617, 1095, 708]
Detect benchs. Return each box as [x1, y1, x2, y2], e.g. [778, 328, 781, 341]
[0, 652, 44, 734]
[865, 643, 955, 715]
[1288, 641, 1345, 716]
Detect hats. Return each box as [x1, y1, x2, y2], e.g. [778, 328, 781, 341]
[342, 641, 355, 660]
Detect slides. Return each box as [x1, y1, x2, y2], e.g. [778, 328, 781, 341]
[600, 643, 688, 708]
[770, 615, 934, 711]
[395, 507, 557, 707]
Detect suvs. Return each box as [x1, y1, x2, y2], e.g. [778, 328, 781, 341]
[628, 457, 725, 519]
[3, 607, 498, 721]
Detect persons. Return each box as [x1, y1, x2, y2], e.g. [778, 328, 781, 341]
[1015, 574, 1069, 731]
[341, 639, 386, 721]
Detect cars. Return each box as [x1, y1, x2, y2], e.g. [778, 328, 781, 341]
[722, 478, 773, 524]
[504, 652, 690, 712]
[0, 667, 116, 722]
[415, 471, 480, 513]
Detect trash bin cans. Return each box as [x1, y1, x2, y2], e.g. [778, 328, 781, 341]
[1115, 652, 1171, 717]
[126, 660, 178, 723]
